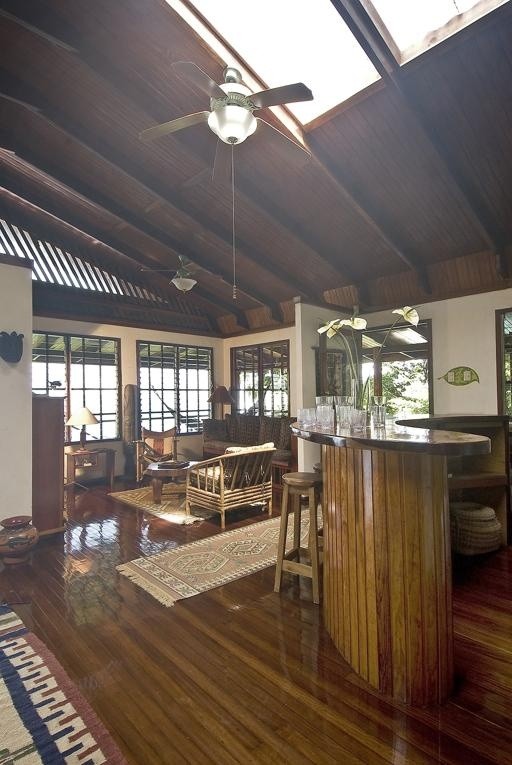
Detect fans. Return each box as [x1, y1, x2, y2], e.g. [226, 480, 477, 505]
[139, 65, 313, 296]
[141, 255, 197, 294]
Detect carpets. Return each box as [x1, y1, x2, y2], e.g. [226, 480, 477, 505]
[116, 504, 323, 608]
[0, 601, 129, 765]
[108, 481, 267, 525]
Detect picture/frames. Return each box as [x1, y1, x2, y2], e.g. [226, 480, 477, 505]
[315, 349, 346, 396]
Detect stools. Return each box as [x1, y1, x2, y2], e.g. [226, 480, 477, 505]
[274, 472, 322, 604]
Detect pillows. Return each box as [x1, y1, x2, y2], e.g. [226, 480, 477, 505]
[218, 442, 274, 483]
[144, 437, 174, 456]
[203, 413, 297, 450]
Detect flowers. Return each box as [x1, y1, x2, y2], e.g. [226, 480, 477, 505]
[318, 307, 419, 410]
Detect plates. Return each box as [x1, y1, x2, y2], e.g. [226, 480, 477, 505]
[158, 461, 191, 469]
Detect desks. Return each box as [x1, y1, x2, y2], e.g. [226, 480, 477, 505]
[64, 446, 118, 489]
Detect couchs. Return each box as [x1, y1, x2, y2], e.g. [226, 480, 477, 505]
[201, 417, 298, 476]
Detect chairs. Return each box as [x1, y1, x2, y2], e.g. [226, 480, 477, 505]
[186, 448, 277, 529]
[133, 426, 177, 482]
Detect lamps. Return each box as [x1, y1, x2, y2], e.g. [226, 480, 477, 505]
[208, 386, 234, 420]
[65, 408, 99, 453]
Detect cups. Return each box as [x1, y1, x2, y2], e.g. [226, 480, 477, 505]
[297, 394, 386, 430]
[321, 429, 385, 441]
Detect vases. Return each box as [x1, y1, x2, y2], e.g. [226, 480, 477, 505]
[0, 516, 39, 565]
[351, 378, 370, 428]
